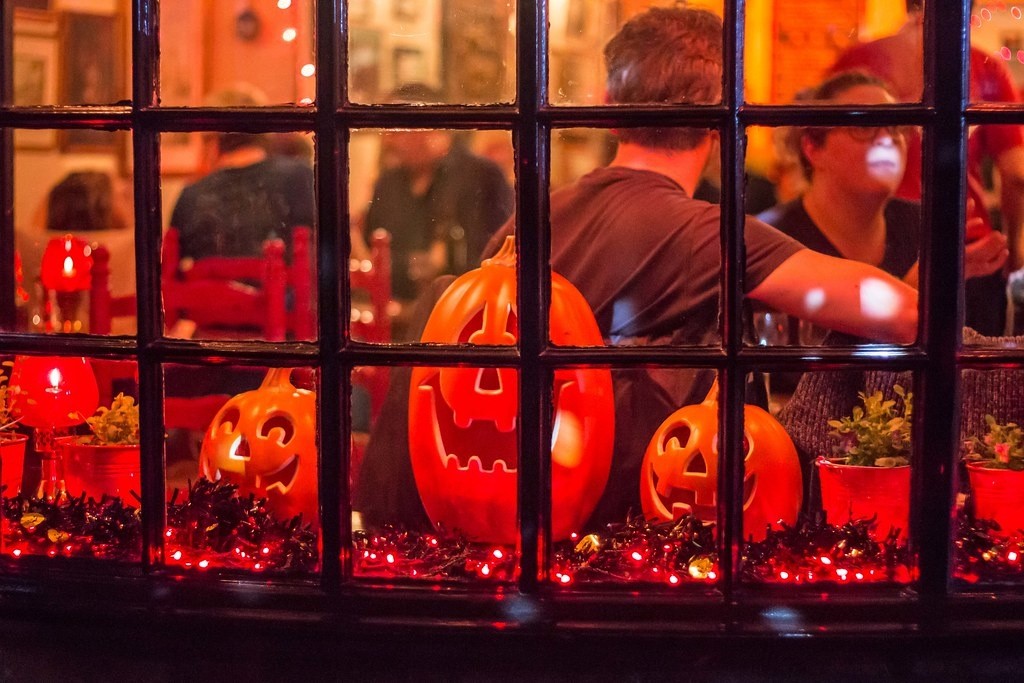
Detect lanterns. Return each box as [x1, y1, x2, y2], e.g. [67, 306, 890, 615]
[408, 264, 615, 542]
[640, 404, 804, 542]
[198, 388, 359, 531]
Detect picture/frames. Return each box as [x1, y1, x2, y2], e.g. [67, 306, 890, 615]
[12, 0, 301, 180]
[347, 0, 601, 192]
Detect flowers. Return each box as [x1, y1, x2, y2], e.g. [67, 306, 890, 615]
[828, 385, 913, 466]
[960, 415, 1024, 472]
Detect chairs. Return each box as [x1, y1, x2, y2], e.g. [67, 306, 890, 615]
[90, 226, 393, 440]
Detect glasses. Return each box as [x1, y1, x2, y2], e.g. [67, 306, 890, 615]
[845, 121, 910, 142]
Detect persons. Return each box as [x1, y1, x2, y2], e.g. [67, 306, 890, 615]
[827, 0, 1024, 337]
[45, 170, 126, 229]
[759, 71, 1009, 338]
[358, 8, 919, 528]
[166, 92, 314, 338]
[364, 83, 511, 299]
[691, 143, 778, 213]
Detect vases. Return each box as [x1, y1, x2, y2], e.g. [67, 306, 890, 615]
[967, 461, 1024, 543]
[815, 457, 911, 552]
